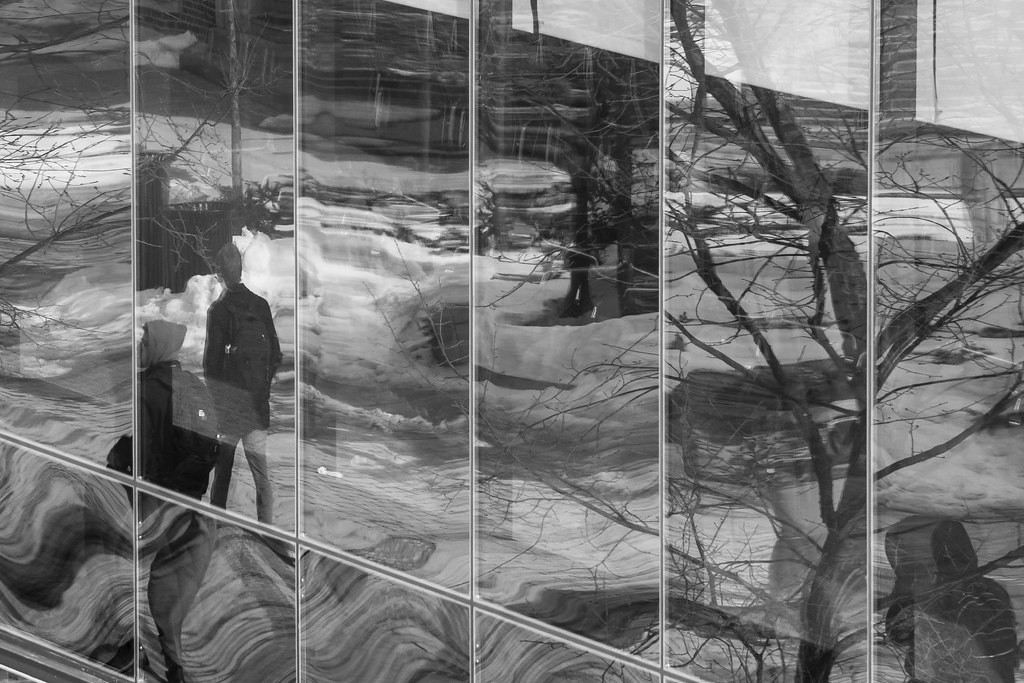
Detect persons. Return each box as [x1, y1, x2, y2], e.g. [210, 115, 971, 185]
[202, 243, 286, 526]
[137, 319, 209, 501]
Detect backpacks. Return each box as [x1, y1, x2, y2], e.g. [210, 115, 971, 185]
[217, 294, 274, 404]
[145, 369, 222, 478]
